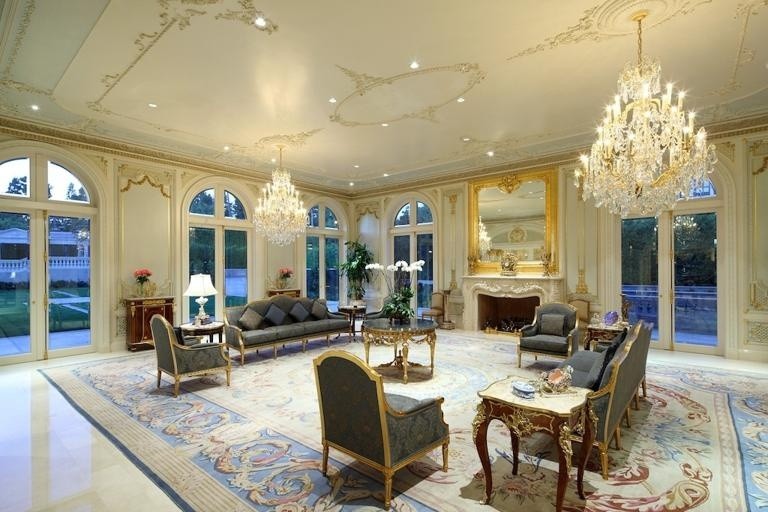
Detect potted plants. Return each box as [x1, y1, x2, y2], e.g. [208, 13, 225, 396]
[339, 236, 374, 306]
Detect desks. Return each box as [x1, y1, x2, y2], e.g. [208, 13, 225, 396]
[471, 373, 599, 512]
[335, 306, 436, 384]
[179, 320, 224, 344]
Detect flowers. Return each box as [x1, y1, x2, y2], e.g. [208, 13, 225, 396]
[279, 267, 293, 278]
[134, 268, 152, 286]
[364, 260, 426, 317]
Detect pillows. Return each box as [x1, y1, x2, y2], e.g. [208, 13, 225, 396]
[238, 300, 327, 330]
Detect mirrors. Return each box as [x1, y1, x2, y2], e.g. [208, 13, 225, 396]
[468, 168, 559, 274]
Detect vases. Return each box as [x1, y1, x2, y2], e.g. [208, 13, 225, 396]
[282, 279, 287, 289]
[137, 286, 146, 295]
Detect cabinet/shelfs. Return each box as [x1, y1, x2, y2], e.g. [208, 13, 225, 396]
[123, 297, 175, 351]
[269, 289, 301, 298]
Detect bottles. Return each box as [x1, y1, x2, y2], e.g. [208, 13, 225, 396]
[590, 313, 599, 327]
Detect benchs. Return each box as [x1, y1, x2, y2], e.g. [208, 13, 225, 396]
[224, 306, 352, 366]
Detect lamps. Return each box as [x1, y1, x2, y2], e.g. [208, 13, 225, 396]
[183, 274, 218, 325]
[251, 145, 310, 247]
[477, 215, 492, 255]
[574, 9, 719, 219]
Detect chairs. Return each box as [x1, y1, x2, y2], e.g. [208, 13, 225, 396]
[149, 313, 230, 397]
[517, 299, 654, 480]
[314, 351, 450, 511]
[422, 293, 445, 322]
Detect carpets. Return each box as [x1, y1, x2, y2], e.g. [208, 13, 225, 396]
[37, 321, 768, 512]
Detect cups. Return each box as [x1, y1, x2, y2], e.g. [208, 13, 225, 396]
[195, 316, 201, 327]
